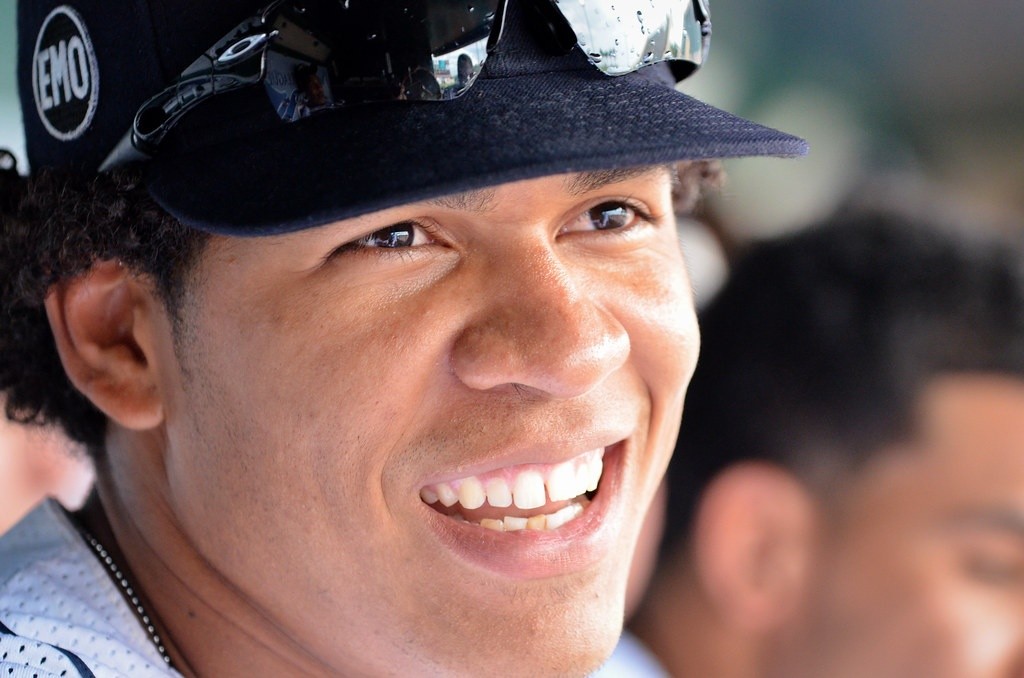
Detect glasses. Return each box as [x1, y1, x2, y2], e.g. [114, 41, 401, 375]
[101, 0, 711, 171]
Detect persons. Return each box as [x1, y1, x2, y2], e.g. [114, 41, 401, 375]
[576, 206, 1024, 678]
[0, 0, 809, 678]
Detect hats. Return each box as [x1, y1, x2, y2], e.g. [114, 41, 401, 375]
[16, 0, 808, 237]
